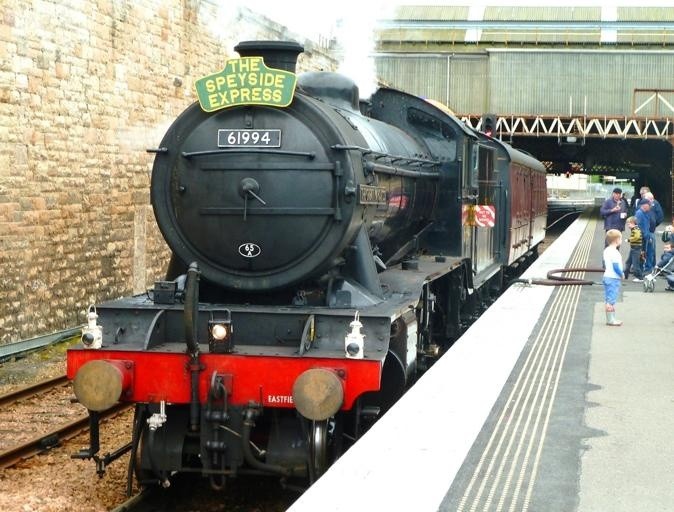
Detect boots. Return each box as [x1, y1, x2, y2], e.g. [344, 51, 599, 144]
[605, 312, 623, 326]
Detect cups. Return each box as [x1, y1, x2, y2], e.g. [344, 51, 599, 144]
[619, 213, 625, 219]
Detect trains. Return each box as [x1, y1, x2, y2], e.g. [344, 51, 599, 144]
[66, 40, 548, 499]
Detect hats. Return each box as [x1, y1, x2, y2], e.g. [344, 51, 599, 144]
[639, 198, 650, 205]
[613, 188, 622, 193]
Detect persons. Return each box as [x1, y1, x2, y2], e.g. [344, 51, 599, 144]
[601, 227, 626, 327]
[596, 186, 674, 294]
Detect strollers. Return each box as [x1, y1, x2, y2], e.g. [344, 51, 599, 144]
[644, 257, 674, 292]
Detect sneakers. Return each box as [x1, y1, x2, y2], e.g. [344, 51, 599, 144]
[632, 278, 644, 283]
